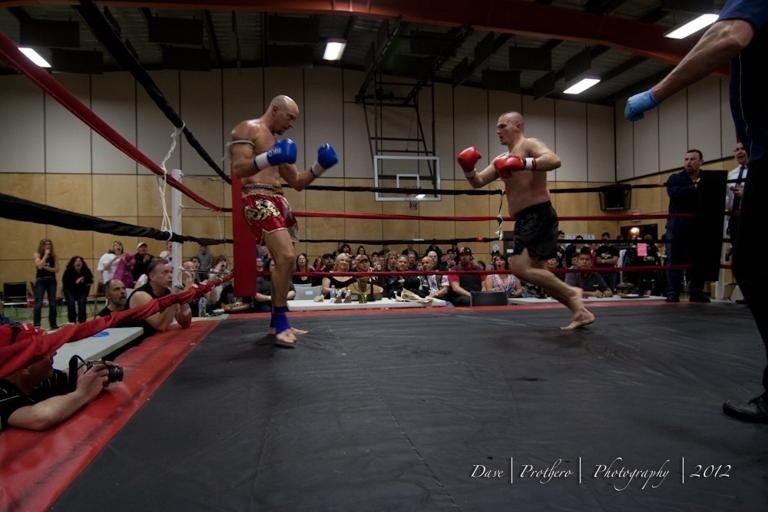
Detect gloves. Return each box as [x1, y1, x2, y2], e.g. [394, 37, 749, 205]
[253, 138, 297, 171]
[310, 143, 338, 178]
[457, 146, 481, 178]
[624, 89, 659, 121]
[495, 155, 536, 180]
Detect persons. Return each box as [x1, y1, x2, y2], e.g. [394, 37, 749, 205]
[665, 149, 710, 303]
[457, 111, 596, 331]
[723, 142, 748, 304]
[230, 94, 339, 344]
[622, 0, 768, 425]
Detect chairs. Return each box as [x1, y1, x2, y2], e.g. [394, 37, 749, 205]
[1, 279, 108, 325]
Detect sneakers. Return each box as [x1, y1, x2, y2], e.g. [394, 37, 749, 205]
[667, 295, 680, 302]
[690, 294, 711, 303]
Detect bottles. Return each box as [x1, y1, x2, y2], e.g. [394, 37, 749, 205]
[199, 297, 206, 316]
[329, 285, 336, 301]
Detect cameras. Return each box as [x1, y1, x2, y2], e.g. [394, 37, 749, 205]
[88, 361, 124, 382]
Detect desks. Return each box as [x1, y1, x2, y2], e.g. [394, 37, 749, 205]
[283, 295, 448, 312]
[506, 294, 668, 306]
[49, 327, 143, 373]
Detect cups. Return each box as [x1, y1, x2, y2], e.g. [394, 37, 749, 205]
[710, 280, 723, 300]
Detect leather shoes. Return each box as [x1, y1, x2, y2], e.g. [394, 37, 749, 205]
[722, 400, 768, 422]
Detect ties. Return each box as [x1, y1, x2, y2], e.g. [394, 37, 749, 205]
[732, 166, 745, 209]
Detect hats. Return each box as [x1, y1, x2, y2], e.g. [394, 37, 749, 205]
[460, 247, 471, 255]
[358, 255, 368, 262]
[0, 322, 45, 345]
[136, 242, 147, 249]
